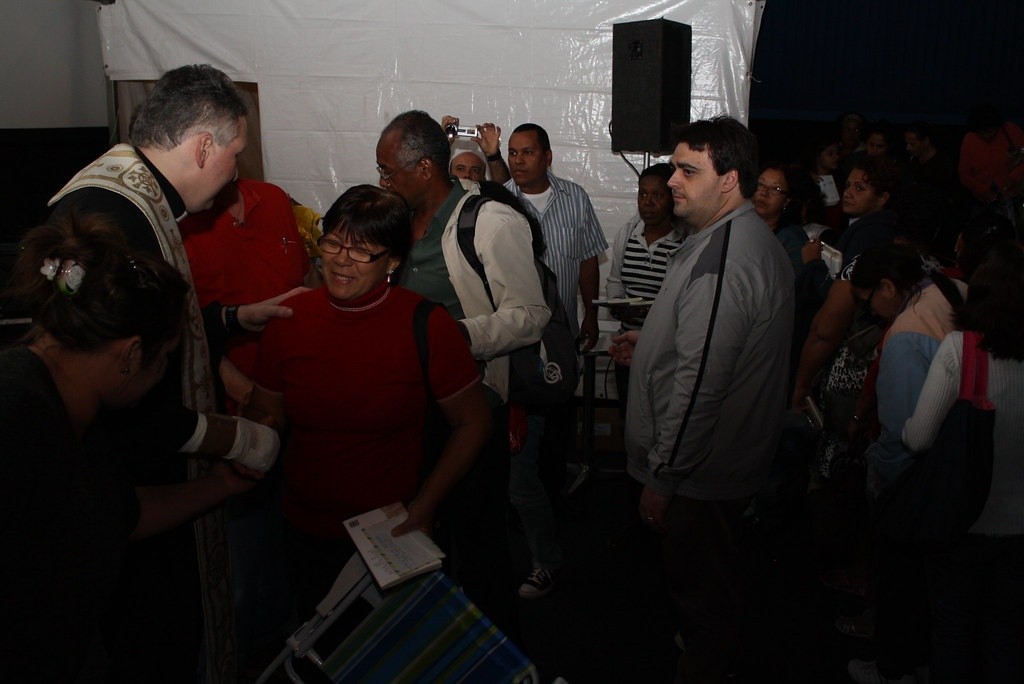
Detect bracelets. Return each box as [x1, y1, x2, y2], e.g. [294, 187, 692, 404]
[486, 149, 501, 161]
[224, 303, 248, 336]
[849, 413, 866, 424]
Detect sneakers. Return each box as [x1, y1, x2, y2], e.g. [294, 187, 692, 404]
[914, 665, 930, 684]
[518, 566, 561, 598]
[847, 659, 916, 684]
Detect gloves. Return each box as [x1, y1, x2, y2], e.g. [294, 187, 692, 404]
[222, 415, 279, 473]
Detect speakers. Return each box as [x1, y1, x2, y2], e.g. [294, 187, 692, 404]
[611, 19, 692, 158]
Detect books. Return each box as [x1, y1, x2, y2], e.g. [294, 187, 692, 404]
[342, 501, 441, 591]
[592, 296, 654, 307]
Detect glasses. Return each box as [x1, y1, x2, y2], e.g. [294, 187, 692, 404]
[317, 231, 391, 263]
[753, 177, 791, 200]
[376, 159, 437, 183]
[856, 280, 885, 315]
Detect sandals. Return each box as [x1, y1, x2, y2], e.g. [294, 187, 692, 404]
[835, 617, 875, 638]
[822, 567, 866, 594]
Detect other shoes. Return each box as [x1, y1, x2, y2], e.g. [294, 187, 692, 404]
[566, 461, 590, 494]
[606, 519, 663, 548]
[674, 630, 739, 678]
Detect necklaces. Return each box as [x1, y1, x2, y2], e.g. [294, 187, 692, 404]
[329, 284, 391, 311]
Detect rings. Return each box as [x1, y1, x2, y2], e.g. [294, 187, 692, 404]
[647, 512, 656, 520]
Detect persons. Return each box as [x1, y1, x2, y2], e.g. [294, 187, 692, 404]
[177, 164, 324, 684]
[252, 181, 493, 619]
[504, 123, 608, 521]
[739, 99, 1024, 684]
[622, 113, 794, 684]
[0, 204, 263, 684]
[371, 110, 555, 638]
[442, 115, 512, 187]
[602, 162, 691, 546]
[46, 61, 314, 684]
[478, 179, 585, 599]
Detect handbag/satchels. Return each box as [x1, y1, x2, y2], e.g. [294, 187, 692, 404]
[506, 399, 529, 457]
[457, 194, 585, 414]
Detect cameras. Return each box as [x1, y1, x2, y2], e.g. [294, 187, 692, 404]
[445, 118, 478, 138]
